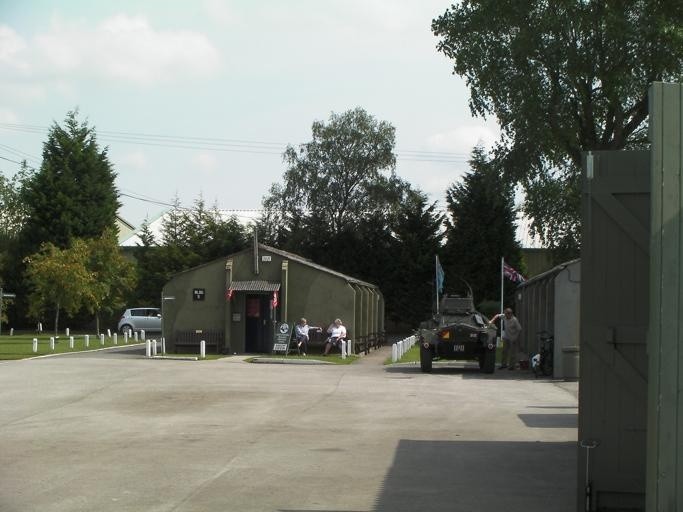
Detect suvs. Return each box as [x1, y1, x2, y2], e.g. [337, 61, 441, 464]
[418, 295, 498, 373]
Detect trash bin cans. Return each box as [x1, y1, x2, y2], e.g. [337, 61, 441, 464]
[562, 346, 580, 381]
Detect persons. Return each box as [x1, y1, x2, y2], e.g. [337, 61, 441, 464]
[489, 308, 523, 370]
[295, 318, 347, 356]
[148, 310, 154, 316]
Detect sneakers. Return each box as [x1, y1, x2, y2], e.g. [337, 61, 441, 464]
[498, 365, 514, 371]
[297, 343, 306, 357]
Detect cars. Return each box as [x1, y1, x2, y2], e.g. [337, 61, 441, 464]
[117, 307, 162, 336]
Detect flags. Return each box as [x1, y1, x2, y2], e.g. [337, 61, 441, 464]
[437, 260, 445, 293]
[503, 263, 525, 283]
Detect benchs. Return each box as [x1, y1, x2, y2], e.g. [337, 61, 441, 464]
[308, 329, 330, 349]
[174, 329, 223, 354]
